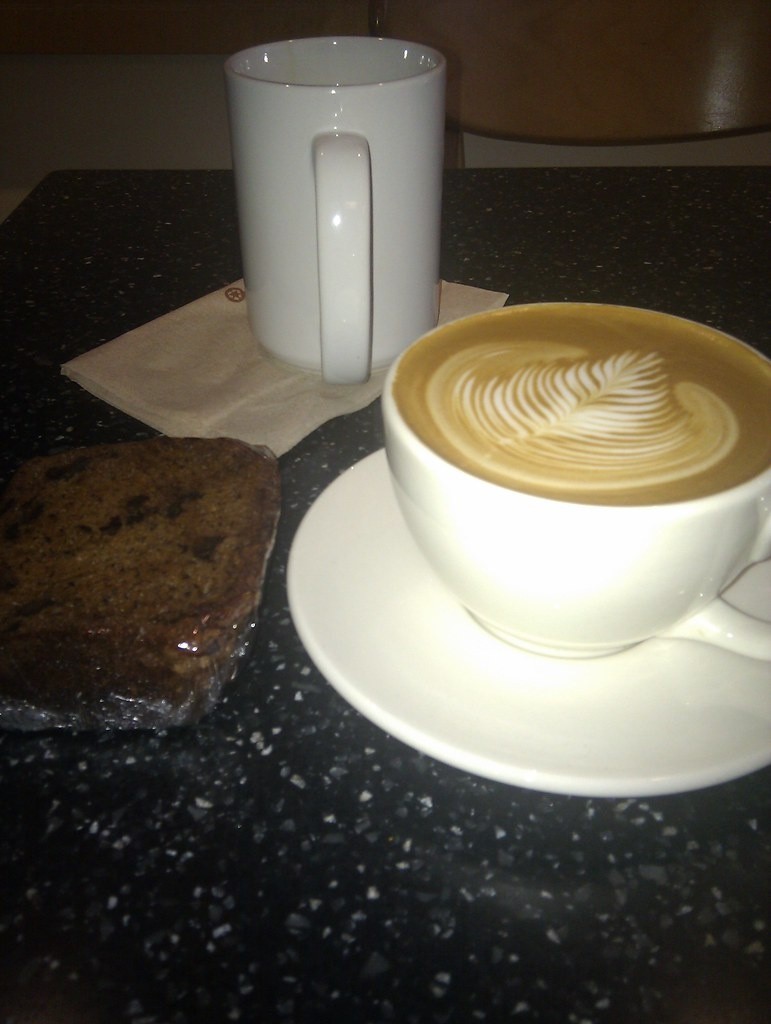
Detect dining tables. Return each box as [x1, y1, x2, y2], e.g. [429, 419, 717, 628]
[1, 160, 771, 1024]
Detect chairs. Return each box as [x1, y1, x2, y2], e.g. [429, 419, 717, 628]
[366, 0, 771, 172]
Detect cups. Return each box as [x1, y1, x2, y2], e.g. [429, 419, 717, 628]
[224, 37, 445, 388]
[383, 300, 771, 656]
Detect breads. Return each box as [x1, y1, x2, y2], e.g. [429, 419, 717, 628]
[0, 437, 281, 705]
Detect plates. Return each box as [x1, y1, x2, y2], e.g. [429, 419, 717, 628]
[288, 443, 771, 797]
